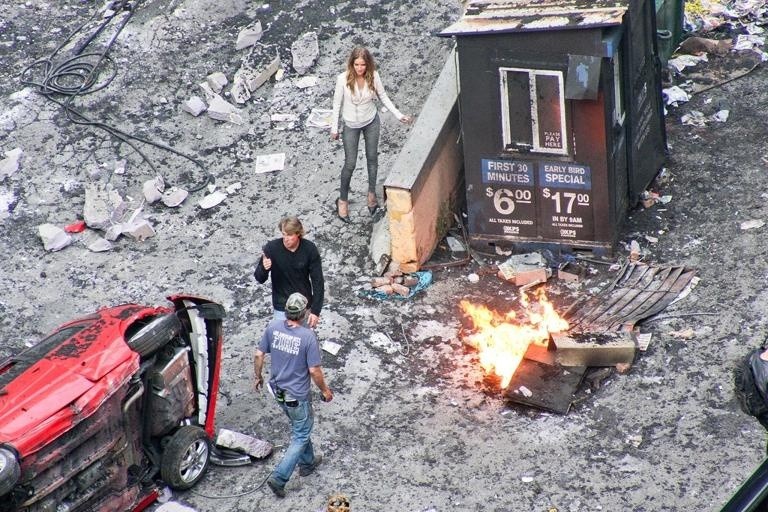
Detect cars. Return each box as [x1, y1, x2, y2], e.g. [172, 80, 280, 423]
[0, 291, 225, 512]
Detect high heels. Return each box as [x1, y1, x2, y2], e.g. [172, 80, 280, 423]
[367, 190, 378, 215]
[335, 197, 350, 223]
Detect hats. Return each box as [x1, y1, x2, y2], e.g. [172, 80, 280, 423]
[284, 291, 308, 313]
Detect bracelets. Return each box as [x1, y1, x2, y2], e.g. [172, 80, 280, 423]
[255, 376, 262, 379]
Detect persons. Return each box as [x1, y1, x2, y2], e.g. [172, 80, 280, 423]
[254, 216, 324, 329]
[330, 47, 411, 223]
[254, 292, 333, 498]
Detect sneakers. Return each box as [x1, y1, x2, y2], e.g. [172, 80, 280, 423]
[299, 455, 323, 476]
[267, 478, 286, 497]
